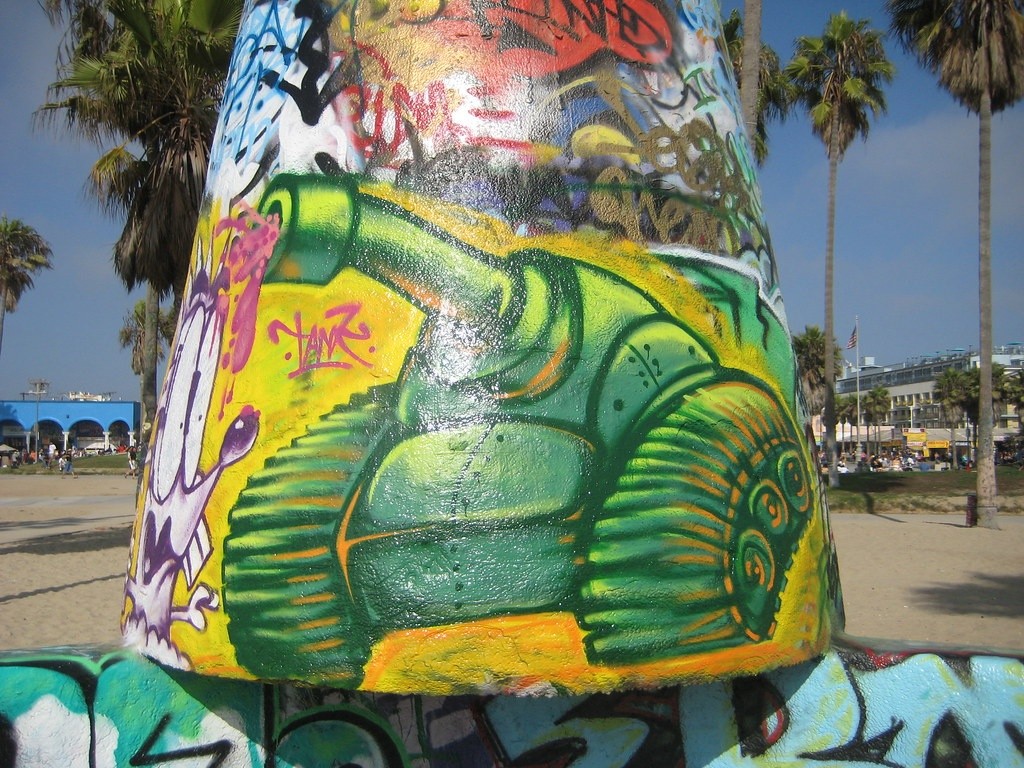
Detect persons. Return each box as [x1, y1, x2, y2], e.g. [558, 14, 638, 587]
[125, 446, 138, 479]
[858, 448, 924, 468]
[11, 450, 20, 468]
[59, 448, 78, 479]
[850, 448, 857, 464]
[837, 460, 849, 473]
[39, 441, 56, 466]
[817, 450, 824, 463]
[934, 451, 939, 459]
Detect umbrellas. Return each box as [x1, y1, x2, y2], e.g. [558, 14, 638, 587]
[0, 444, 17, 452]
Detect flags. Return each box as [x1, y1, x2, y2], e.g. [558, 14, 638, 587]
[846, 323, 856, 349]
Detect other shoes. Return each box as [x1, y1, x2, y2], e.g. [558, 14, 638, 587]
[124, 474, 127, 478]
[132, 476, 137, 479]
[46, 466, 54, 471]
[73, 476, 78, 479]
[62, 477, 67, 479]
